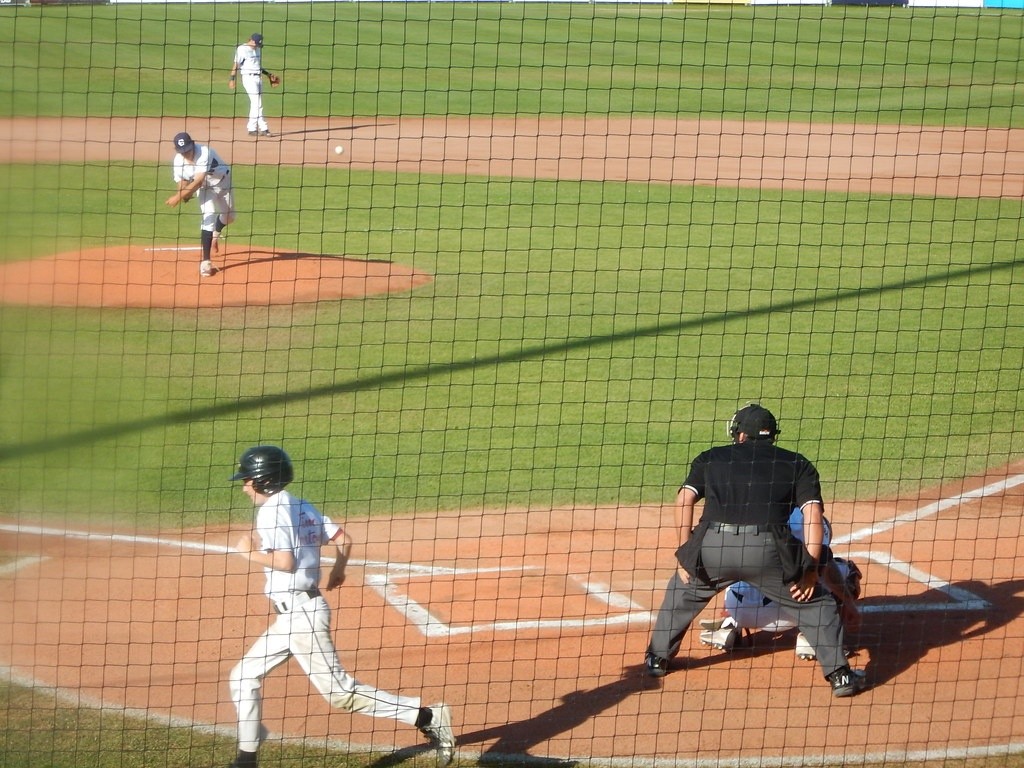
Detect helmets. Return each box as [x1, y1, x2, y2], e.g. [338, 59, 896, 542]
[228, 446, 294, 497]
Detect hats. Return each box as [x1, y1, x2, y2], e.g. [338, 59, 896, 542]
[734, 405, 782, 439]
[252, 33, 263, 48]
[174, 132, 193, 154]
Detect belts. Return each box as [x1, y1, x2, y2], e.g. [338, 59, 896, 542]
[732, 590, 772, 605]
[249, 73, 261, 76]
[273, 586, 322, 615]
[699, 520, 790, 535]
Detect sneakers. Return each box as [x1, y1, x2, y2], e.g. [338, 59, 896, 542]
[700, 628, 736, 649]
[420, 702, 455, 766]
[829, 668, 866, 697]
[795, 632, 854, 659]
[645, 649, 669, 677]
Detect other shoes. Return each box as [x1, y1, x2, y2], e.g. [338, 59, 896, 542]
[248, 130, 261, 136]
[210, 237, 218, 253]
[261, 130, 270, 136]
[201, 260, 212, 277]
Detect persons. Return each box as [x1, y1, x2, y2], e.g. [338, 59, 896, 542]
[229, 33, 279, 137]
[229, 446, 455, 768]
[645, 403, 869, 698]
[165, 132, 235, 277]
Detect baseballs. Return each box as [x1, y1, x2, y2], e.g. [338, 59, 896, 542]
[335, 146, 343, 155]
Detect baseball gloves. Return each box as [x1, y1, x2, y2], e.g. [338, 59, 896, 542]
[268, 73, 280, 88]
[833, 557, 863, 604]
